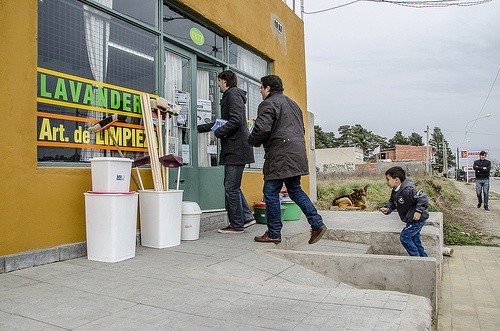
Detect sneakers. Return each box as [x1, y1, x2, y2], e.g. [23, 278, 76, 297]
[218, 225, 244, 233]
[244, 219, 257, 229]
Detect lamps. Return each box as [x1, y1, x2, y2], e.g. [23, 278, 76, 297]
[108, 40, 155, 62]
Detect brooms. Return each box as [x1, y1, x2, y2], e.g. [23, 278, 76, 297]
[92, 113, 140, 189]
[151, 97, 166, 191]
[131, 156, 151, 190]
[156, 97, 182, 191]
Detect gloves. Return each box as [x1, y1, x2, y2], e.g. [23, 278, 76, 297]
[213, 127, 224, 139]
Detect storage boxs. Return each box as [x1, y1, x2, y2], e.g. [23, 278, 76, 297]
[83, 157, 185, 263]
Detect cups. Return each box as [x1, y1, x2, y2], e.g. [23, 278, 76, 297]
[443, 246, 454, 257]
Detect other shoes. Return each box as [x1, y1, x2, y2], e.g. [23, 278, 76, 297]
[477, 202, 481, 208]
[484, 205, 490, 211]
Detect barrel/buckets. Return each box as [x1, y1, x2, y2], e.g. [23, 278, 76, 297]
[181, 214, 201, 241]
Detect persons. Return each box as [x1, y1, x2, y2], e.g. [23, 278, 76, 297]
[197, 70, 256, 234]
[474, 151, 491, 210]
[248, 75, 327, 244]
[379, 167, 429, 257]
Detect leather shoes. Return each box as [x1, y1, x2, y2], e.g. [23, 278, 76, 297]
[307, 223, 327, 244]
[254, 231, 281, 243]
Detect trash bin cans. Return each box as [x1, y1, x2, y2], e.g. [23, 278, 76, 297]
[181, 201, 202, 240]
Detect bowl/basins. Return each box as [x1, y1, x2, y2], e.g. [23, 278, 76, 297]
[181, 201, 202, 215]
[281, 202, 301, 220]
[253, 205, 286, 224]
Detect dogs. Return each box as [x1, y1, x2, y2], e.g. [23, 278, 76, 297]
[331, 185, 368, 211]
